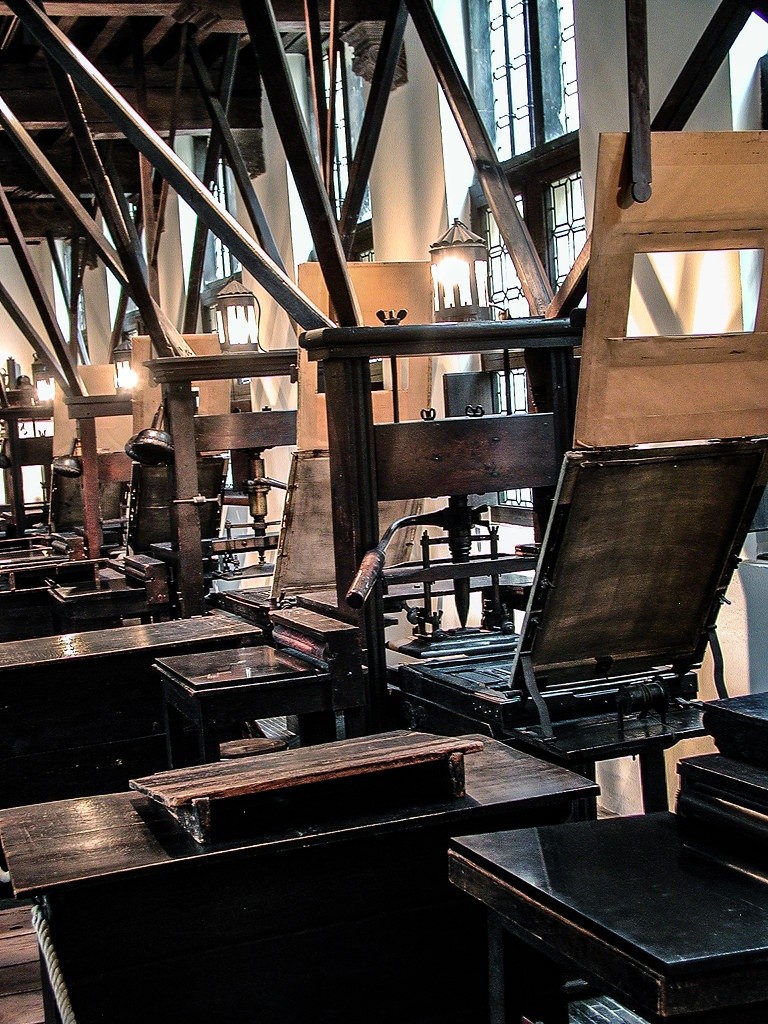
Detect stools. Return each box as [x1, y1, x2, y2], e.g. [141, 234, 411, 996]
[48, 582, 145, 631]
[153, 645, 328, 765]
[446, 810, 768, 1024]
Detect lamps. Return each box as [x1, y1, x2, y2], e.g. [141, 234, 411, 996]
[113, 331, 132, 395]
[36, 370, 55, 405]
[428, 218, 493, 323]
[218, 275, 267, 354]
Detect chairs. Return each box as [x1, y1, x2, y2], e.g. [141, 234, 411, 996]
[101, 456, 229, 579]
[219, 450, 422, 646]
[399, 435, 767, 814]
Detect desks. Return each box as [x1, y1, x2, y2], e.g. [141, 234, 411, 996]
[0, 731, 600, 1024]
[0, 615, 263, 668]
